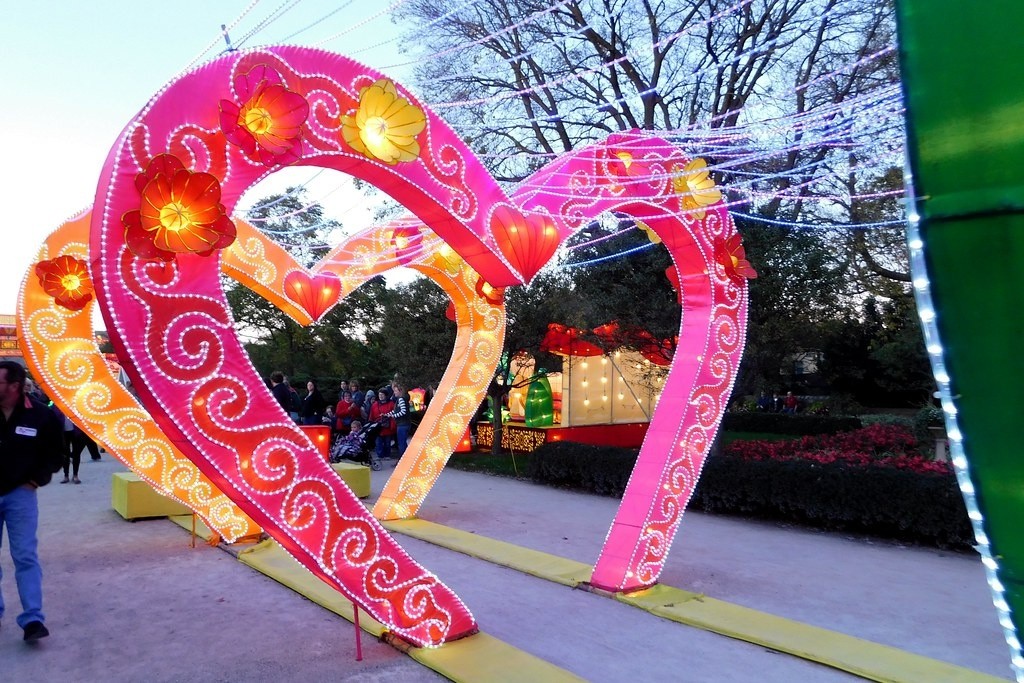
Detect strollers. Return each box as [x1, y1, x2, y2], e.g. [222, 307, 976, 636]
[329, 415, 390, 471]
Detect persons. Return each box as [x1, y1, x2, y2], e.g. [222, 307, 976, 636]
[263, 371, 412, 467]
[0, 361, 107, 640]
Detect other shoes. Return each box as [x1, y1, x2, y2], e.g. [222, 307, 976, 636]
[60, 478, 69, 483]
[385, 457, 390, 460]
[73, 477, 81, 483]
[24, 621, 49, 640]
[393, 458, 400, 467]
[375, 457, 382, 461]
[101, 449, 105, 453]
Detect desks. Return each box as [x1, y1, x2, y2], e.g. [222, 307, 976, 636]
[111, 473, 194, 519]
[332, 463, 372, 499]
